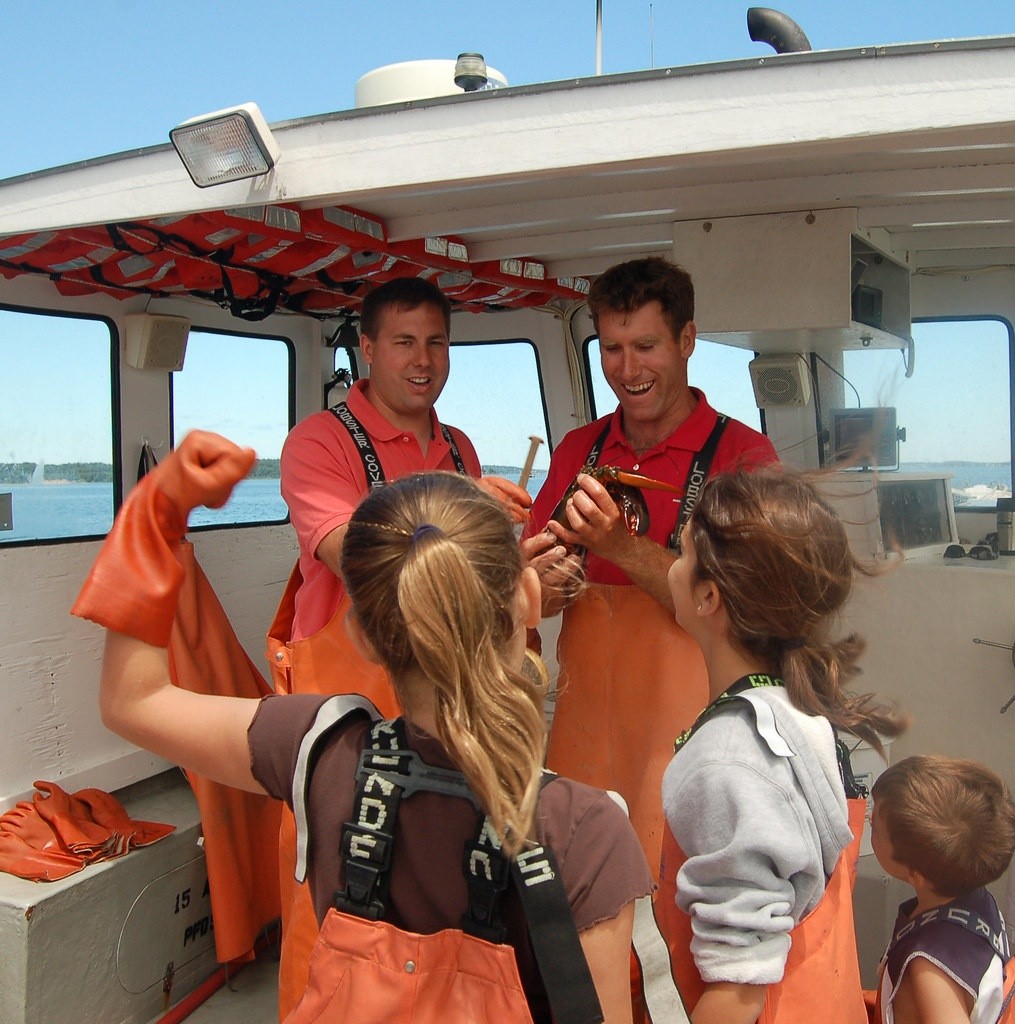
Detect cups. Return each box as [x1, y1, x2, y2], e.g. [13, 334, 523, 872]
[997, 498, 1015, 556]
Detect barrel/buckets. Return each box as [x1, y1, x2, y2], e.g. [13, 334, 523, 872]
[837, 726, 895, 856]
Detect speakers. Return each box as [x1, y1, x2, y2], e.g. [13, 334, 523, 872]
[748, 350, 810, 410]
[124, 312, 190, 374]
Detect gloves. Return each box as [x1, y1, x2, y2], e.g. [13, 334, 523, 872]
[0, 780, 177, 881]
[70, 428, 259, 648]
[474, 476, 533, 524]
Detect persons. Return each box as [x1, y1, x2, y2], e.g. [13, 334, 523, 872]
[518, 260, 792, 1024]
[280, 277, 482, 1018]
[656, 469, 911, 1024]
[72, 429, 654, 1024]
[870, 754, 1015, 1024]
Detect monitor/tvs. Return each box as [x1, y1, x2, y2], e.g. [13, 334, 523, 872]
[875, 477, 962, 561]
